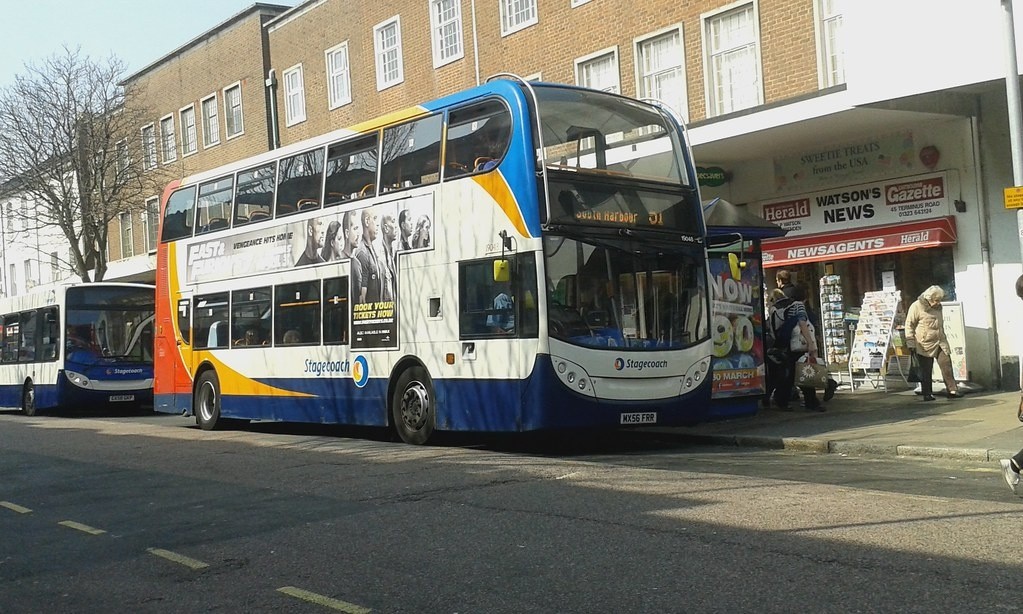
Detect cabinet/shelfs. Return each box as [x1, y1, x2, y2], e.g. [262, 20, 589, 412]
[820, 277, 849, 384]
[849, 290, 911, 393]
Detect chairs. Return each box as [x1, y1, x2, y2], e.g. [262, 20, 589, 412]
[447, 155, 493, 178]
[208, 185, 401, 232]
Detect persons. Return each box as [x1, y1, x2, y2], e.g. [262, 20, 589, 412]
[486, 292, 515, 334]
[245, 317, 310, 347]
[294, 208, 431, 305]
[761, 269, 837, 412]
[904, 285, 964, 401]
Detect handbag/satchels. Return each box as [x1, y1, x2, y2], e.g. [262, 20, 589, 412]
[793, 351, 828, 389]
[789, 313, 816, 352]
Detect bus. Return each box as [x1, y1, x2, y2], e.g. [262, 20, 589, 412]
[152, 72, 746, 447]
[0, 279, 163, 418]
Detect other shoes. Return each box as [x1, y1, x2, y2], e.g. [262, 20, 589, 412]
[924, 393, 935, 400]
[948, 390, 964, 398]
[812, 404, 826, 411]
[774, 399, 792, 411]
[1000, 458, 1020, 494]
[823, 379, 837, 401]
